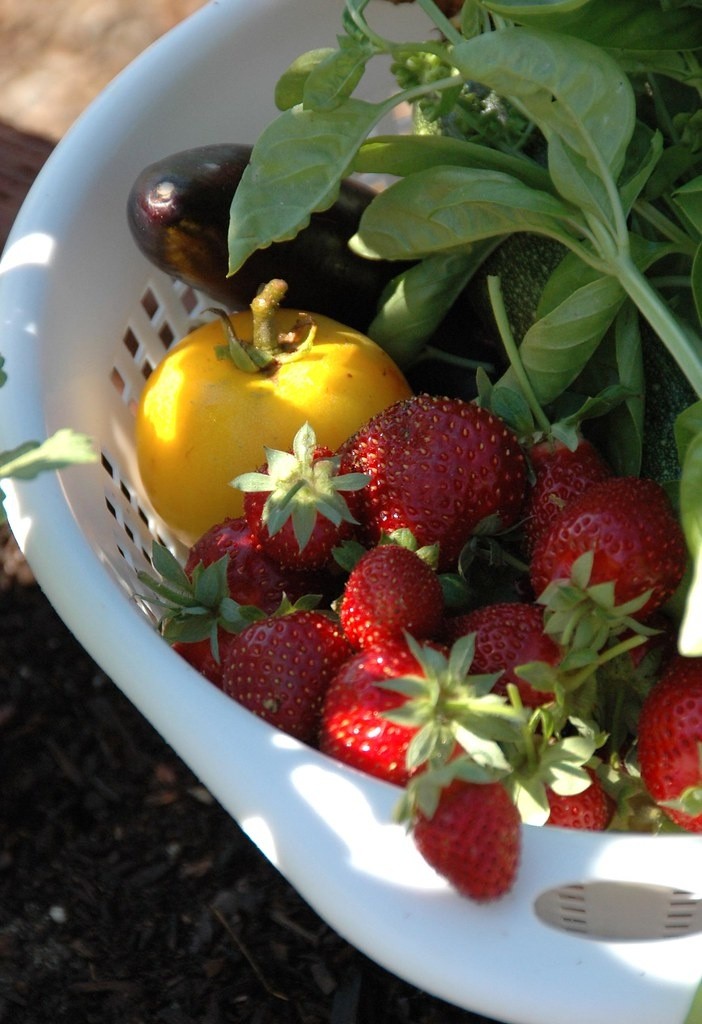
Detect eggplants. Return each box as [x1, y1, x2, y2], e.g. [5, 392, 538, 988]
[126, 146, 505, 401]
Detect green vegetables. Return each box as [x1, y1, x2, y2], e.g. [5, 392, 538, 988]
[221, 0, 702, 659]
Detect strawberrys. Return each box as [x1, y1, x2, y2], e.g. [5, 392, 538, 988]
[132, 276, 702, 901]
